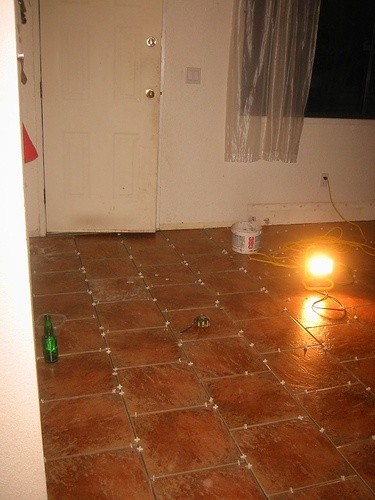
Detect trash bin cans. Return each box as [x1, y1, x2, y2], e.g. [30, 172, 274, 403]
[230, 221, 263, 255]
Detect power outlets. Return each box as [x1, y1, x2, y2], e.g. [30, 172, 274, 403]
[321, 173, 328, 188]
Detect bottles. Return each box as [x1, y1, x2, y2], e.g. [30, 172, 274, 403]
[43, 314, 59, 364]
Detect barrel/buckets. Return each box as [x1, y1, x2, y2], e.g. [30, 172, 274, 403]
[230, 220, 264, 255]
